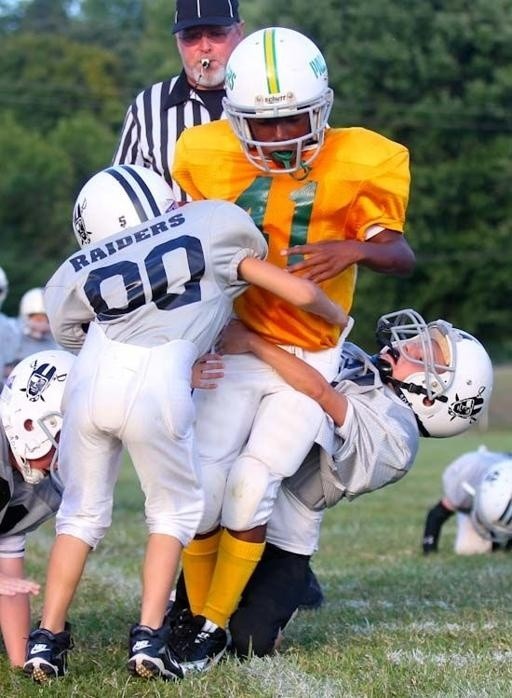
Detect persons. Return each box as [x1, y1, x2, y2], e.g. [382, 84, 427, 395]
[168, 27, 415, 671]
[0, 350, 78, 672]
[0, 265, 21, 391]
[422, 450, 512, 556]
[18, 286, 66, 373]
[168, 307, 495, 661]
[23, 164, 349, 681]
[112, 1, 326, 609]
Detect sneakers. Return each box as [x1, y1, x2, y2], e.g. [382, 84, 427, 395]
[299, 566, 322, 610]
[24, 622, 71, 685]
[165, 591, 175, 613]
[128, 626, 184, 681]
[163, 607, 191, 643]
[170, 617, 226, 675]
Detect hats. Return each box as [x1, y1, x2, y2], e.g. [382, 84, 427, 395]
[171, 0, 240, 35]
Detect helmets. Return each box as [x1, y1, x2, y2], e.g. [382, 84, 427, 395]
[0, 267, 9, 311]
[222, 28, 333, 174]
[378, 310, 493, 439]
[74, 167, 180, 251]
[1, 350, 79, 484]
[472, 460, 511, 542]
[19, 287, 53, 339]
